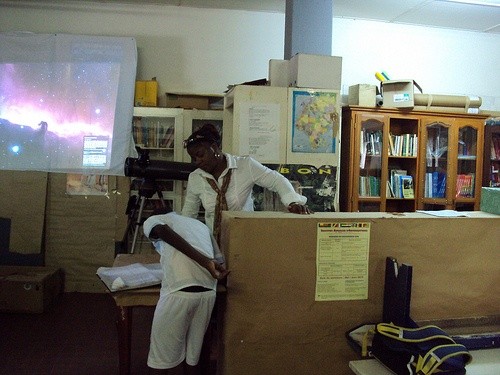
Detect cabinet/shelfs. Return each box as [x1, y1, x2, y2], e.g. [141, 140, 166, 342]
[223, 85, 342, 214]
[127, 107, 223, 244]
[339, 105, 500, 213]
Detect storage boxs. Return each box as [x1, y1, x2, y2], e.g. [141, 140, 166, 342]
[347, 83, 379, 109]
[1, 265, 65, 313]
[133, 79, 158, 109]
[268, 54, 342, 89]
[379, 79, 423, 109]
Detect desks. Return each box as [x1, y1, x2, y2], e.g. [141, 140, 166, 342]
[105, 254, 227, 375]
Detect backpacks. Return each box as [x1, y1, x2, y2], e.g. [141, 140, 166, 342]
[369, 321, 473, 375]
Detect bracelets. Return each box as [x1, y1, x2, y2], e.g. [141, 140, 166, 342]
[291, 203, 299, 206]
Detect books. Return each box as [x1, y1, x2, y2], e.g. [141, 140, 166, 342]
[133, 118, 174, 148]
[359, 128, 418, 157]
[424, 172, 475, 198]
[358, 166, 414, 199]
[489, 137, 500, 187]
[427, 126, 468, 155]
[96, 263, 164, 293]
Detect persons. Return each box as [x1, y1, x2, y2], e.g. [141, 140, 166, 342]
[180, 124, 316, 248]
[142, 207, 231, 375]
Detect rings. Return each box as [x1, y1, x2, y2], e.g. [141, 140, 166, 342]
[305, 205, 308, 208]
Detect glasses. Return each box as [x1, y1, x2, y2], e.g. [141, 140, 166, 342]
[183, 135, 210, 149]
